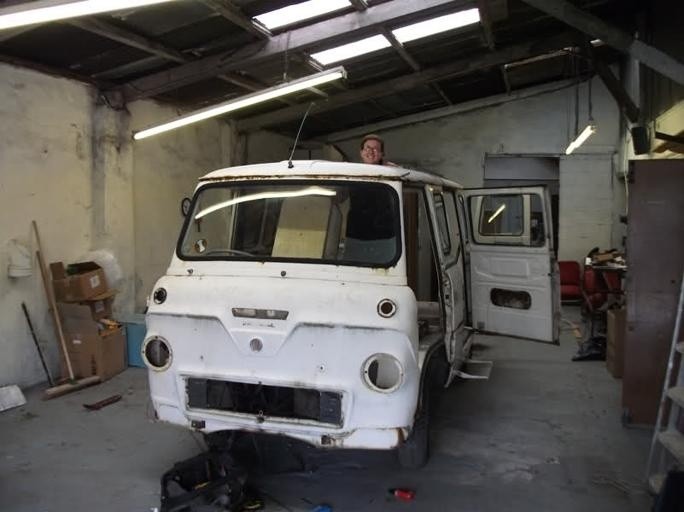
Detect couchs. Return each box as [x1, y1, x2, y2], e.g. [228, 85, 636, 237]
[558, 260, 585, 307]
[580, 268, 622, 317]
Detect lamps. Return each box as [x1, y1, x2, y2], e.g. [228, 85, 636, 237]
[0, 0, 179, 32]
[564, 34, 598, 157]
[131, 31, 348, 142]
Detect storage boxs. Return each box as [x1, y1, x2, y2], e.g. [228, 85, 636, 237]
[49, 259, 107, 300]
[54, 317, 129, 383]
[605, 342, 624, 378]
[116, 310, 148, 369]
[56, 287, 123, 321]
[606, 308, 628, 355]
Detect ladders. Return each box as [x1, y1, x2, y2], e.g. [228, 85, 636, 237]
[644, 279, 684, 495]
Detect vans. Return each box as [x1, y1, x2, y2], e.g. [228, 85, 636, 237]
[140, 160, 559, 469]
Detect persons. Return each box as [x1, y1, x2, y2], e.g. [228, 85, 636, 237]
[331, 136, 401, 264]
